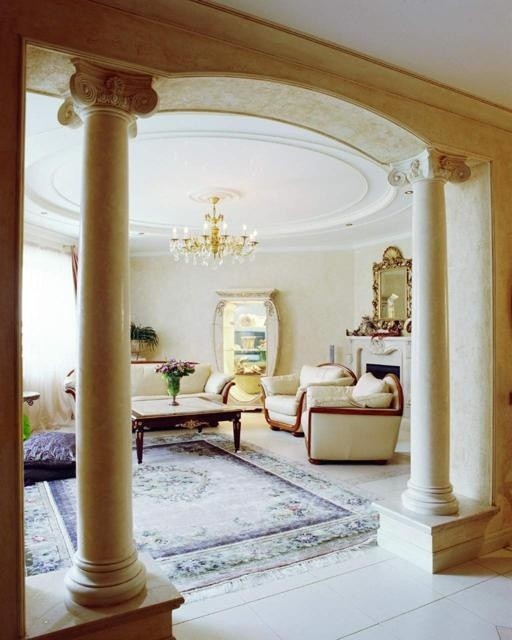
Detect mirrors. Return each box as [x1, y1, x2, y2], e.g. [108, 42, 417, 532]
[372, 247, 412, 332]
[213, 288, 279, 411]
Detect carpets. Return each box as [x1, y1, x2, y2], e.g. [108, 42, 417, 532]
[23, 432, 380, 595]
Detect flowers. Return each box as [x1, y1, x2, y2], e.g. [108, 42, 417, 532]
[155, 362, 196, 396]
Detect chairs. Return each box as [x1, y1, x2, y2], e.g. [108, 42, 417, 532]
[258, 363, 357, 437]
[301, 374, 404, 465]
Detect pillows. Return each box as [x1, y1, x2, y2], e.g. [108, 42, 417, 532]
[24, 432, 76, 466]
[204, 373, 235, 393]
[260, 364, 394, 408]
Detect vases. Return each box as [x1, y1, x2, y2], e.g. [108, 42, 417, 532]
[167, 377, 179, 406]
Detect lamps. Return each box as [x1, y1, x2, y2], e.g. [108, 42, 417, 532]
[170, 197, 258, 265]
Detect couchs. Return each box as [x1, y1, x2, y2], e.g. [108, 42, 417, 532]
[64, 361, 236, 432]
